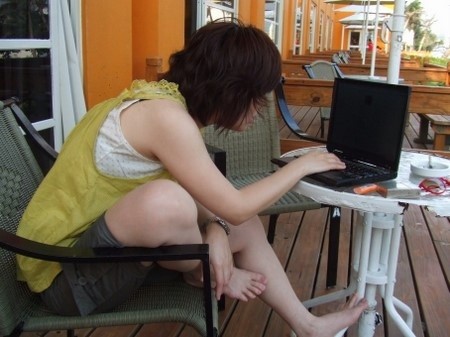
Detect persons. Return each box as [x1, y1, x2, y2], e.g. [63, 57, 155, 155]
[15, 17, 367, 337]
[367, 39, 372, 52]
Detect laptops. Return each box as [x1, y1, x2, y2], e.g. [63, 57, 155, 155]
[270, 77, 412, 188]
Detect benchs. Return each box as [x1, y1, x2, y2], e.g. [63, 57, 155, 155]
[416, 114, 450, 151]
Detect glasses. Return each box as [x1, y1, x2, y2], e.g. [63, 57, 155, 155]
[418, 176, 450, 195]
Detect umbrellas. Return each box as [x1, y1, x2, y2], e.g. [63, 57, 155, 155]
[325, 0, 393, 75]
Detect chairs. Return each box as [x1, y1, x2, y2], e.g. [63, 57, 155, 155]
[200, 81, 342, 311]
[303, 50, 354, 138]
[0, 97, 218, 337]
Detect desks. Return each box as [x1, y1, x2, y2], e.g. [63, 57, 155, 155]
[278, 146, 450, 337]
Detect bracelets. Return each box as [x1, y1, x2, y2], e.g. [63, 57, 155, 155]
[201, 216, 231, 236]
